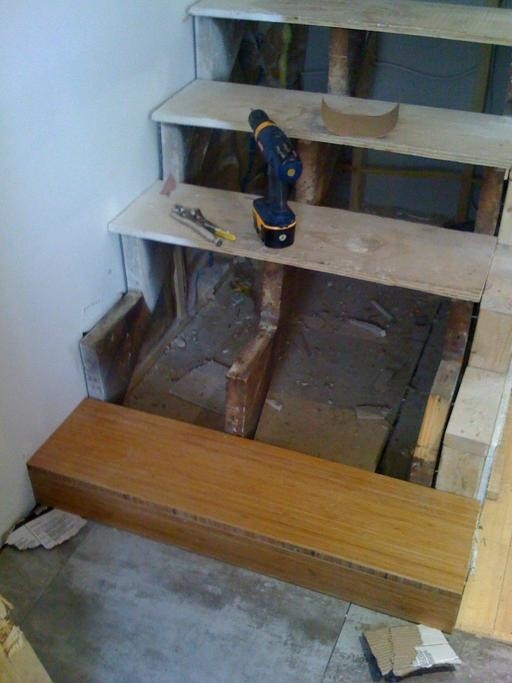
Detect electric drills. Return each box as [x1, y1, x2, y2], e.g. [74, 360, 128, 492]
[248, 106, 303, 249]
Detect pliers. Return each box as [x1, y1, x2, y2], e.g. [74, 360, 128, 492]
[174, 204, 236, 246]
